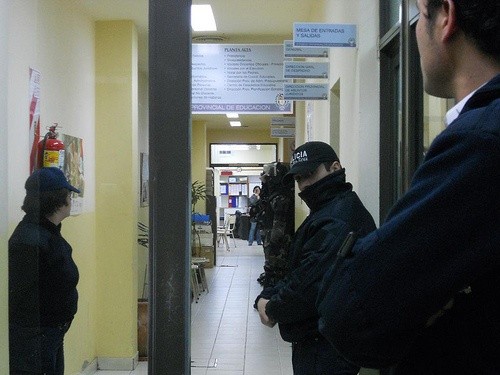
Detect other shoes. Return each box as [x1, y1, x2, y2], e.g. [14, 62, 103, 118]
[258, 243, 263, 245]
[249, 243, 252, 246]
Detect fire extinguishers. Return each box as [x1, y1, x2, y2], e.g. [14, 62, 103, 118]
[38, 123, 65, 170]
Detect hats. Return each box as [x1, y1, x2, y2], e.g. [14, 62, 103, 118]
[26, 167, 81, 193]
[283, 141, 340, 180]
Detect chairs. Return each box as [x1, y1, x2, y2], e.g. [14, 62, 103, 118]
[217, 214, 236, 252]
[191, 257, 210, 303]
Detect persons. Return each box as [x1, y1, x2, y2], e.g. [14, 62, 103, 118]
[248, 162, 293, 290]
[8, 168, 81, 375]
[319, 0, 499, 375]
[254, 141, 378, 375]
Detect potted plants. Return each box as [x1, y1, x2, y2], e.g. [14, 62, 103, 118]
[137, 221, 148, 362]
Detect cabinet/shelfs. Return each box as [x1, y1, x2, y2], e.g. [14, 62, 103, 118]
[228, 176, 249, 208]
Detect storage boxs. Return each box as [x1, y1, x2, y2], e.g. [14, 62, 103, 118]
[192, 225, 214, 268]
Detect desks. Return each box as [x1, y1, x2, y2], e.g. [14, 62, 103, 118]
[230, 214, 257, 241]
